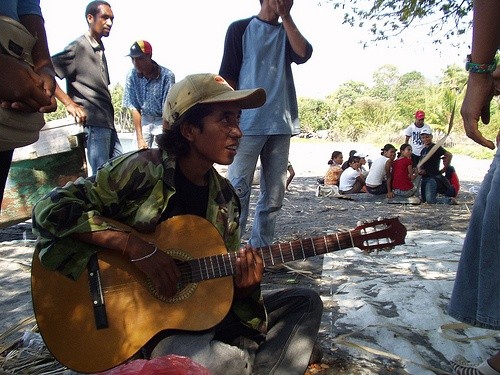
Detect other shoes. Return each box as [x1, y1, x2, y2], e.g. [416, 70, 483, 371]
[264, 263, 285, 272]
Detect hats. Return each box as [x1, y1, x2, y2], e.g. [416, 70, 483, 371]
[420, 128, 432, 135]
[126, 40, 152, 57]
[415, 110, 425, 119]
[163, 73, 267, 126]
[354, 152, 368, 158]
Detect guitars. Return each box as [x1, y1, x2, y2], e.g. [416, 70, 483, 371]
[30, 214, 406, 374]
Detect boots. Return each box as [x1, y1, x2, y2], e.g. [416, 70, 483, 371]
[450, 359, 499, 375]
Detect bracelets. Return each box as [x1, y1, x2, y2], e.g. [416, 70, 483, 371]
[122, 234, 132, 254]
[130, 246, 157, 261]
[466, 62, 496, 73]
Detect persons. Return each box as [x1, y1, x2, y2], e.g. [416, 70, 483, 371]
[364, 143, 396, 199]
[339, 150, 369, 194]
[446, 0, 500, 375]
[123, 40, 174, 149]
[33, 73, 324, 375]
[323, 151, 344, 185]
[418, 128, 460, 205]
[218, 0, 312, 268]
[49, 0, 124, 176]
[0, 0, 57, 211]
[404, 110, 432, 161]
[392, 144, 421, 197]
[286, 161, 295, 189]
